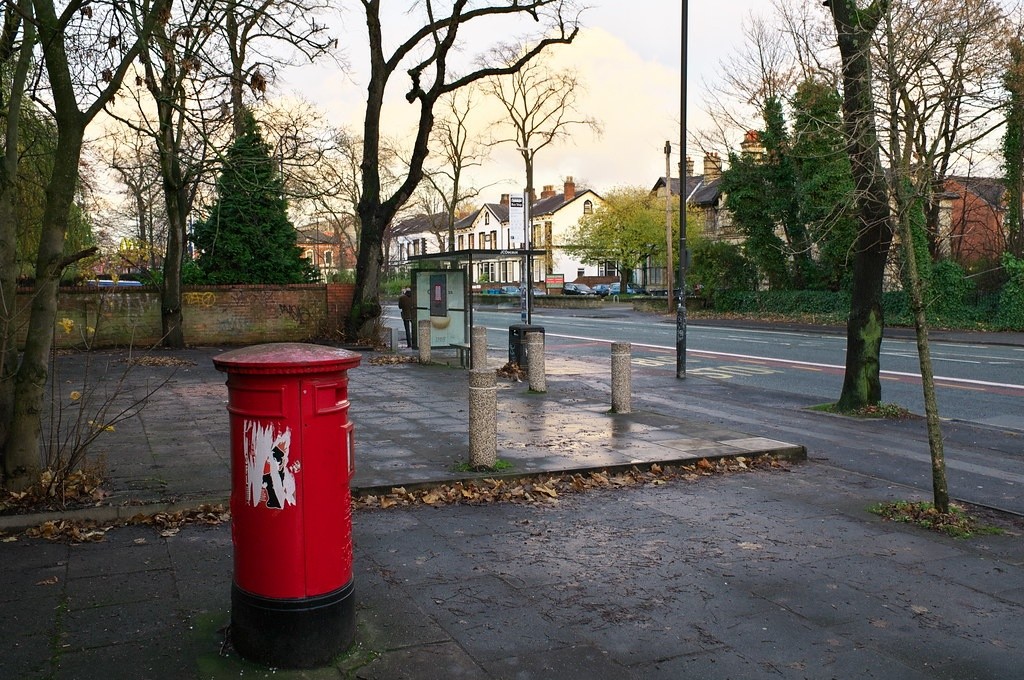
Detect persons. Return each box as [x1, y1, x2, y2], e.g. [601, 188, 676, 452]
[397, 284, 418, 350]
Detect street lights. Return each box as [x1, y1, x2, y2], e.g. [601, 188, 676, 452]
[277, 131, 302, 206]
[516, 148, 535, 324]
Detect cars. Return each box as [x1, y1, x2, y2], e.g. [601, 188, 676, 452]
[498, 286, 521, 294]
[562, 283, 596, 295]
[593, 284, 610, 297]
[609, 281, 648, 294]
[519, 285, 545, 295]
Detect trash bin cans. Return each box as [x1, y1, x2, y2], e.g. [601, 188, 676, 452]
[508, 325, 544, 373]
